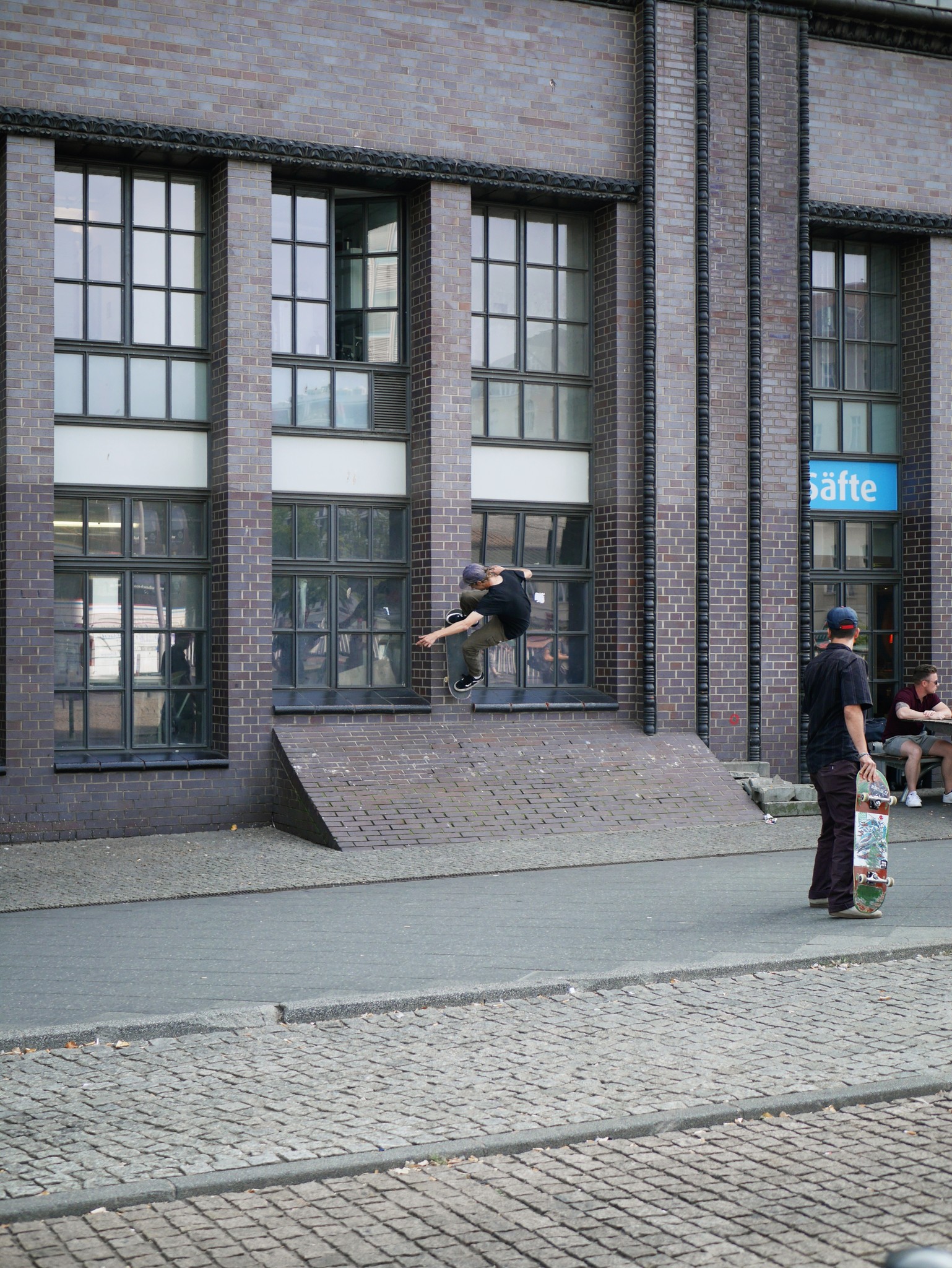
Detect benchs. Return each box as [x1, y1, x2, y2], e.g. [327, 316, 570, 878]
[870, 750, 946, 803]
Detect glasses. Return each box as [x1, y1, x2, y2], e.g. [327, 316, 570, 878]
[922, 678, 939, 685]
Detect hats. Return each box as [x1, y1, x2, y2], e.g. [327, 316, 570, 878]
[459, 563, 486, 589]
[822, 606, 858, 631]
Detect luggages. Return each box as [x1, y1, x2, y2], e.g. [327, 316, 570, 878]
[889, 767, 923, 790]
[867, 741, 887, 780]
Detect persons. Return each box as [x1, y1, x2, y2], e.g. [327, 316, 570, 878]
[155, 633, 196, 740]
[542, 640, 569, 686]
[804, 605, 885, 918]
[415, 563, 532, 691]
[882, 663, 952, 808]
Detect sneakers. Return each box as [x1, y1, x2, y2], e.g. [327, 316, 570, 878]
[942, 789, 952, 803]
[454, 672, 485, 692]
[905, 794, 922, 807]
[446, 613, 479, 627]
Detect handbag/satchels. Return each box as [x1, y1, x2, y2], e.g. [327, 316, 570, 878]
[865, 717, 887, 742]
[527, 641, 554, 673]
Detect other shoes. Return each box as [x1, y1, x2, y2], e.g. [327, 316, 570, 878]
[829, 904, 883, 918]
[809, 897, 829, 908]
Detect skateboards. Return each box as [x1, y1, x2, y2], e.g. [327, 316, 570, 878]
[443, 608, 472, 700]
[853, 770, 897, 914]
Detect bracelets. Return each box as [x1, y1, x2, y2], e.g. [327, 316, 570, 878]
[857, 753, 870, 759]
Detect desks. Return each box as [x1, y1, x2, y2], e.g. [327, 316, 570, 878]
[906, 716, 952, 741]
